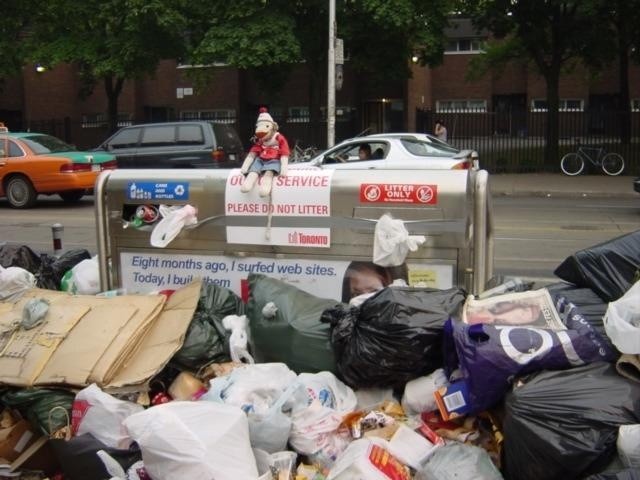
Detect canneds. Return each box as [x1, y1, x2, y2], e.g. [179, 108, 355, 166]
[135, 205, 155, 221]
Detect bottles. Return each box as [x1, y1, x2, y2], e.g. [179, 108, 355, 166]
[474, 277, 534, 303]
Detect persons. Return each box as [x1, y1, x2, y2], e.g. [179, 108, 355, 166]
[336, 146, 371, 163]
[435, 119, 448, 142]
[341, 262, 390, 303]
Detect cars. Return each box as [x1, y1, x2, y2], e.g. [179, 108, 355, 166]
[287, 131, 479, 169]
[0, 120, 245, 209]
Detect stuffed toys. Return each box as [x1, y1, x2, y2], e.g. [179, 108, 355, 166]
[240, 106, 292, 197]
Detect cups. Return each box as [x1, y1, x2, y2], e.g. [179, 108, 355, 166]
[267, 450, 297, 478]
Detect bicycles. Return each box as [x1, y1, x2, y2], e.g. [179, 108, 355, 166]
[560, 138, 625, 176]
